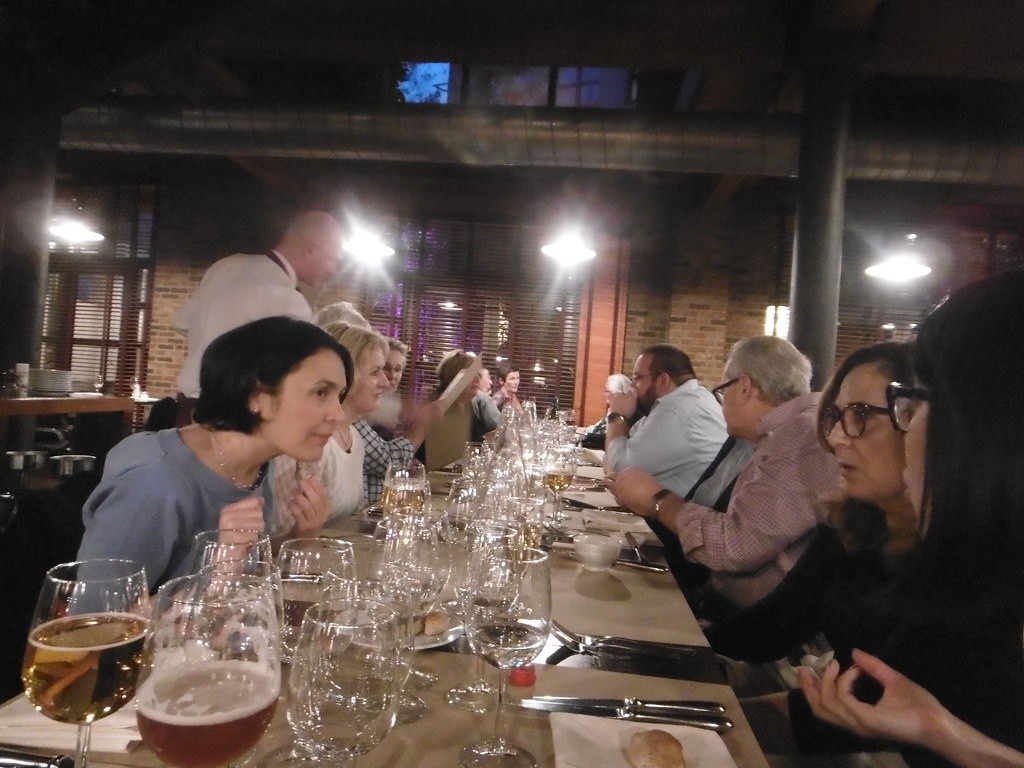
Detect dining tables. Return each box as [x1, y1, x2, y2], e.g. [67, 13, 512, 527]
[0, 427, 771, 768]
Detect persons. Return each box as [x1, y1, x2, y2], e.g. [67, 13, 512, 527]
[172, 207, 345, 427]
[69, 303, 525, 620]
[601, 272, 1024, 768]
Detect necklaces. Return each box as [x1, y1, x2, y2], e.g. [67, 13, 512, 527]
[337, 430, 351, 451]
[211, 434, 264, 490]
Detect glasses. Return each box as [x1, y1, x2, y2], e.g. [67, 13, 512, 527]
[885, 379, 936, 434]
[629, 371, 672, 385]
[713, 375, 740, 407]
[821, 401, 894, 439]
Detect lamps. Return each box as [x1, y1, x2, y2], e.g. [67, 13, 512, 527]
[48, 179, 105, 243]
[539, 180, 597, 261]
[864, 208, 932, 282]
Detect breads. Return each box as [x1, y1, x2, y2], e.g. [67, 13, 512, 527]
[628, 728, 687, 768]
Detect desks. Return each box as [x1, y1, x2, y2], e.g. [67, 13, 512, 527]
[0, 392, 135, 531]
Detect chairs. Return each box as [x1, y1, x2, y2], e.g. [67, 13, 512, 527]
[1, 469, 105, 706]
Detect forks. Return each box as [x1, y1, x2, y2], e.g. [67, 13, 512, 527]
[550, 619, 707, 660]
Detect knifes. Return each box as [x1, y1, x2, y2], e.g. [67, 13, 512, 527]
[502, 694, 735, 732]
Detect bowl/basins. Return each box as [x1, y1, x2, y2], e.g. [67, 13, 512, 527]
[573, 534, 620, 571]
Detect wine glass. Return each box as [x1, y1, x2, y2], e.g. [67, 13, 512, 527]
[21, 401, 578, 768]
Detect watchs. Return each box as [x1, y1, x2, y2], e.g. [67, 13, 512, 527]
[606, 412, 627, 425]
[651, 488, 672, 519]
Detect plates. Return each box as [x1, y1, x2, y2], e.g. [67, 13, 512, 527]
[549, 710, 737, 768]
[335, 605, 462, 651]
